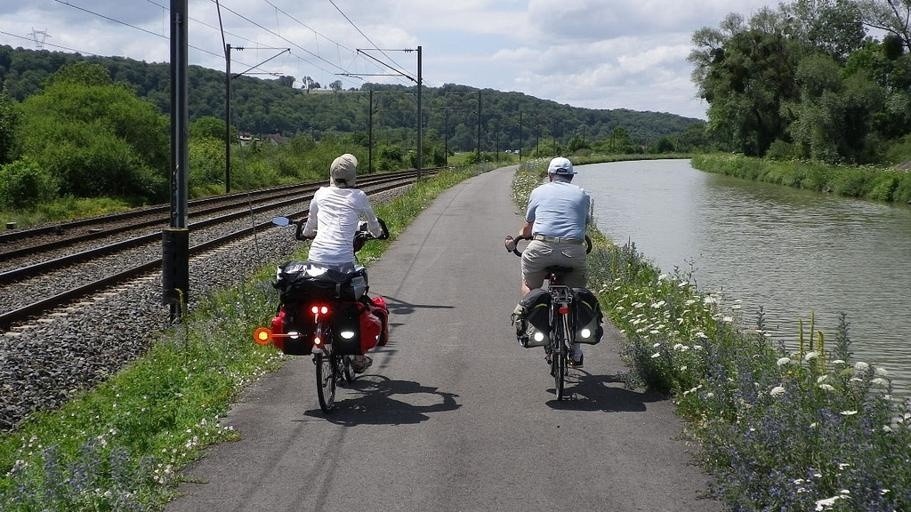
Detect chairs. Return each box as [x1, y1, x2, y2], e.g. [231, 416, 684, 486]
[352, 357, 372, 373]
[569, 353, 583, 368]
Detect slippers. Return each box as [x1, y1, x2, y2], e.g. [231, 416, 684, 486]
[572, 287, 603, 345]
[511, 288, 550, 348]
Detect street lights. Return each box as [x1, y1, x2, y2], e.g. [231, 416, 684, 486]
[273, 261, 368, 301]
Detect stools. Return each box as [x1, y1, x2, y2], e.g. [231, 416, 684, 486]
[533, 235, 583, 245]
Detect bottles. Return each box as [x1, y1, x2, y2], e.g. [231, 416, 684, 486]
[547, 157, 576, 175]
[330, 154, 358, 187]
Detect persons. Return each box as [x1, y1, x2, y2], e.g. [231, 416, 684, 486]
[302, 153, 382, 373]
[520, 157, 594, 367]
